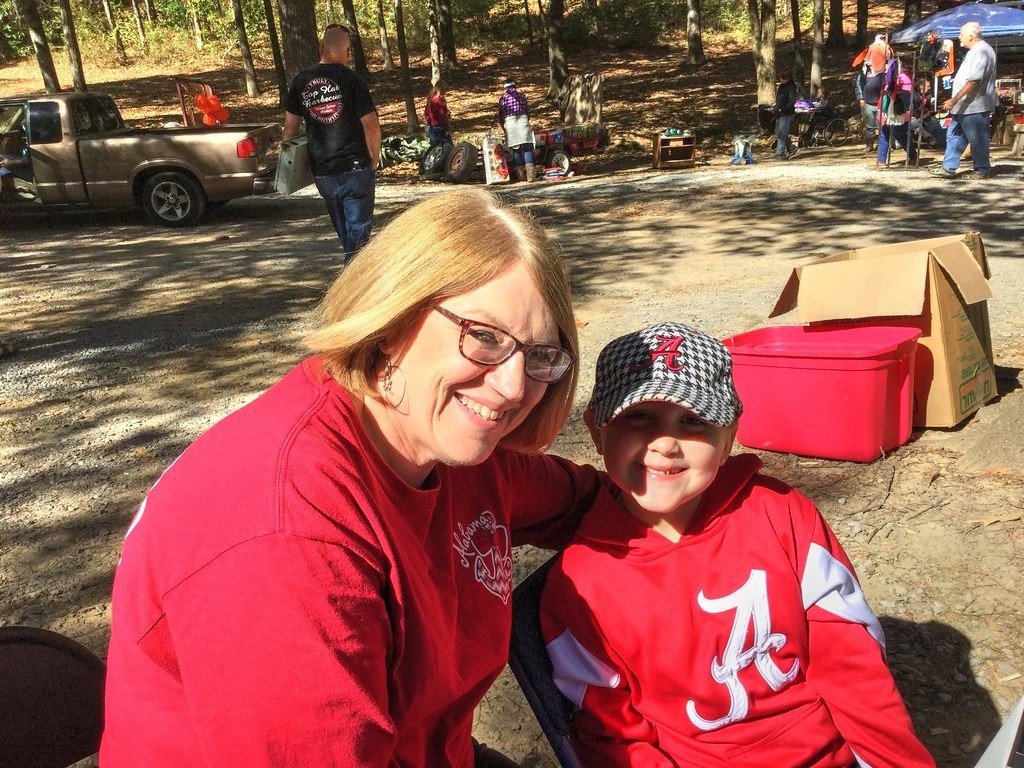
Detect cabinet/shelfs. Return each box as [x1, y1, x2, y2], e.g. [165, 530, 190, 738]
[653, 133, 697, 169]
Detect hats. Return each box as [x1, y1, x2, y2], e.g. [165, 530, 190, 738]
[586, 323, 744, 429]
[503, 78, 516, 89]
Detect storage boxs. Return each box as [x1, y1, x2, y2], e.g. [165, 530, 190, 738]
[482, 136, 510, 185]
[719, 327, 922, 462]
[769, 228, 998, 428]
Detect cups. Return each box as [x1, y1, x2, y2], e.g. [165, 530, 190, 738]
[942, 76, 951, 89]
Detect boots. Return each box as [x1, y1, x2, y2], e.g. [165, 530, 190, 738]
[526, 163, 539, 182]
[862, 139, 873, 153]
[515, 165, 527, 180]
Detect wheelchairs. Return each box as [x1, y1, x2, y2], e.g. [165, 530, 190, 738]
[794, 114, 851, 148]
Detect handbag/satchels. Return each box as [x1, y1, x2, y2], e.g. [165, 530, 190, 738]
[894, 90, 920, 115]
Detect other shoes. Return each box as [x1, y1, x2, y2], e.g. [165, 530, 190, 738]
[903, 158, 917, 165]
[929, 166, 955, 177]
[775, 153, 787, 162]
[867, 160, 886, 169]
[962, 168, 992, 180]
[788, 146, 800, 160]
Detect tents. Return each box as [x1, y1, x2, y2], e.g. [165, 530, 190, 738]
[891, 1, 1024, 112]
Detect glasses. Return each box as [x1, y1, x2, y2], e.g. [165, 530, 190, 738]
[326, 24, 350, 38]
[426, 299, 579, 384]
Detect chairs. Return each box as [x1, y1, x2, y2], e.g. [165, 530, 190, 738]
[508, 548, 589, 768]
[0, 625, 105, 768]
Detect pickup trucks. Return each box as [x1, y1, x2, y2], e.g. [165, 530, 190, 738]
[0, 92, 285, 230]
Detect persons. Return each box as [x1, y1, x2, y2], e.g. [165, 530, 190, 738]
[768, 70, 801, 160]
[856, 68, 945, 170]
[283, 27, 381, 266]
[801, 87, 833, 142]
[0, 155, 33, 197]
[498, 80, 539, 181]
[541, 322, 936, 768]
[425, 80, 451, 144]
[98, 190, 604, 767]
[928, 22, 996, 178]
